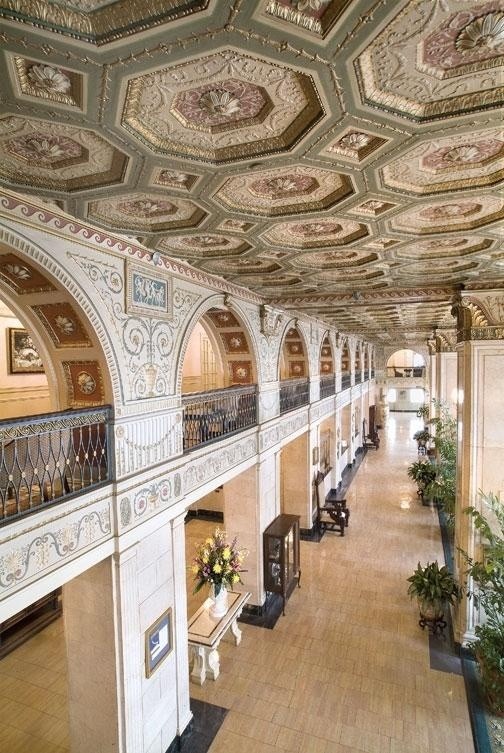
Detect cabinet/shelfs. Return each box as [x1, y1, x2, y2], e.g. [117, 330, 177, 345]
[263, 514, 302, 617]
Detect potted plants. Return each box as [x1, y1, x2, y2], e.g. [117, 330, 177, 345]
[419, 479, 435, 506]
[407, 460, 436, 491]
[444, 488, 504, 721]
[413, 430, 431, 455]
[405, 558, 462, 635]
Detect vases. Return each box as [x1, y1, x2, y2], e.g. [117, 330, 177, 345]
[374, 395, 389, 429]
[208, 583, 228, 618]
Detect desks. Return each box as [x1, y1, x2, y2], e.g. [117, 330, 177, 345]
[182, 407, 228, 450]
[187, 590, 252, 686]
[404, 369, 412, 377]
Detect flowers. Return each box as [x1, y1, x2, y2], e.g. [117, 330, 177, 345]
[185, 525, 249, 598]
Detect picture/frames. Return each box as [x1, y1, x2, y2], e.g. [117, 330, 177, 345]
[125, 259, 174, 320]
[9, 327, 45, 373]
[313, 447, 319, 465]
[144, 607, 174, 679]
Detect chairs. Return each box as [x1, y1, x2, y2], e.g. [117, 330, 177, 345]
[393, 366, 402, 377]
[362, 418, 379, 451]
[314, 466, 350, 542]
[0, 407, 72, 502]
[413, 369, 422, 377]
